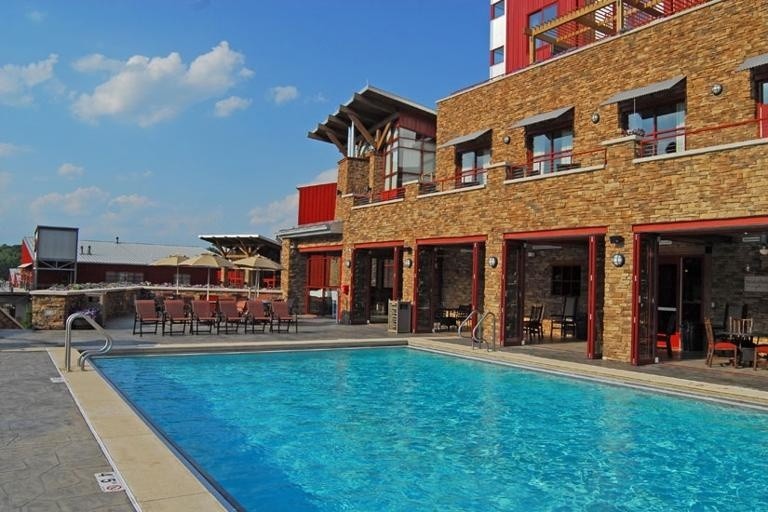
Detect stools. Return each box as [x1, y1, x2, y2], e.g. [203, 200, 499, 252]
[439, 316, 459, 332]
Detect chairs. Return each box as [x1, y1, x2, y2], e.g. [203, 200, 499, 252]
[549, 296, 580, 341]
[523, 305, 545, 340]
[656, 322, 676, 359]
[133, 299, 165, 336]
[244, 300, 273, 334]
[269, 301, 298, 333]
[161, 300, 193, 336]
[189, 300, 220, 335]
[217, 300, 247, 334]
[703, 313, 768, 371]
[456, 303, 472, 332]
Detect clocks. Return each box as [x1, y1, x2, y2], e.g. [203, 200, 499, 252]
[711, 83, 722, 96]
[345, 260, 351, 268]
[406, 259, 412, 268]
[503, 136, 510, 144]
[488, 257, 497, 268]
[590, 112, 599, 124]
[611, 254, 624, 267]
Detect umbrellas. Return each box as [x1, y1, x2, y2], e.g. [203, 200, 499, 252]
[178, 253, 234, 302]
[147, 255, 188, 296]
[234, 254, 288, 299]
